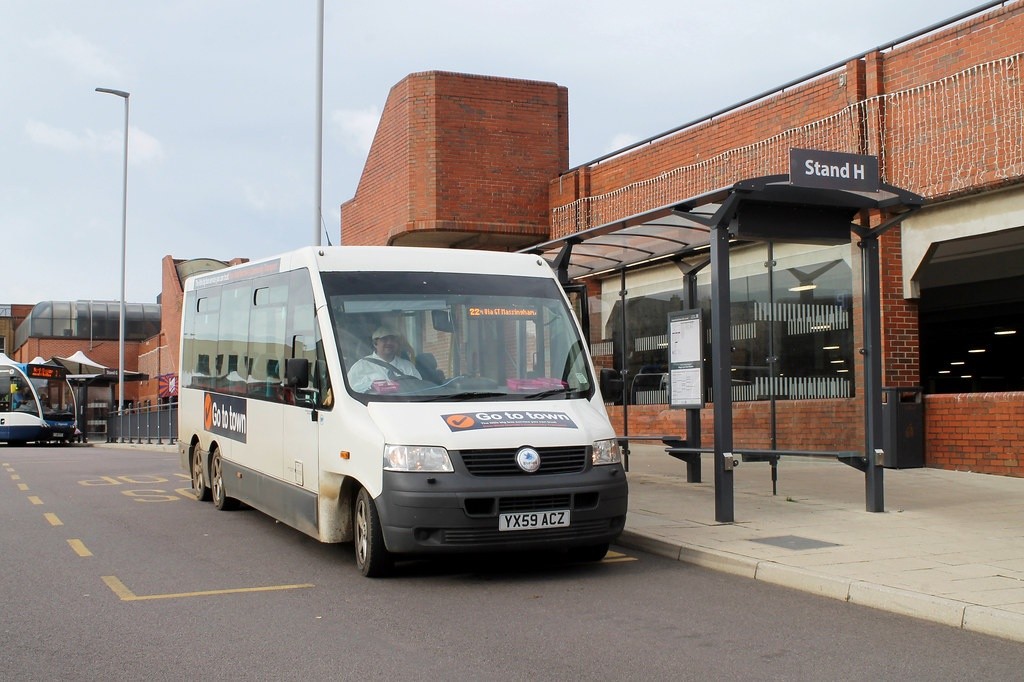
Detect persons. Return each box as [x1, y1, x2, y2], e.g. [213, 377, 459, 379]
[13, 384, 32, 411]
[347, 325, 422, 393]
[776, 361, 855, 398]
[35, 394, 76, 443]
[123, 409, 130, 416]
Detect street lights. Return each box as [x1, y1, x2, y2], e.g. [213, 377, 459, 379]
[92, 86, 132, 444]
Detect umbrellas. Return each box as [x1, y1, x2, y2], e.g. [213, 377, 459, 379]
[0, 350, 142, 381]
[191, 371, 282, 386]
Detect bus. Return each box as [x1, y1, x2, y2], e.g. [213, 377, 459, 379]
[0, 361, 78, 446]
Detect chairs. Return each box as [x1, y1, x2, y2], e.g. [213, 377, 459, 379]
[415, 352, 444, 385]
[356, 338, 376, 361]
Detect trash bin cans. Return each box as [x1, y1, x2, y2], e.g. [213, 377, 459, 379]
[880, 385, 925, 468]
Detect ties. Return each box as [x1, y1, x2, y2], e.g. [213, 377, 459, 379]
[388, 371, 395, 381]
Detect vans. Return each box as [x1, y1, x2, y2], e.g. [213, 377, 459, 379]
[177, 245, 630, 579]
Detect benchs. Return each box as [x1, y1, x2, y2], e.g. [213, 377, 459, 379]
[618, 436, 687, 448]
[88, 432, 107, 436]
[666, 447, 866, 472]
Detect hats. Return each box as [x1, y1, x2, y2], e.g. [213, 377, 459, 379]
[372, 326, 397, 339]
